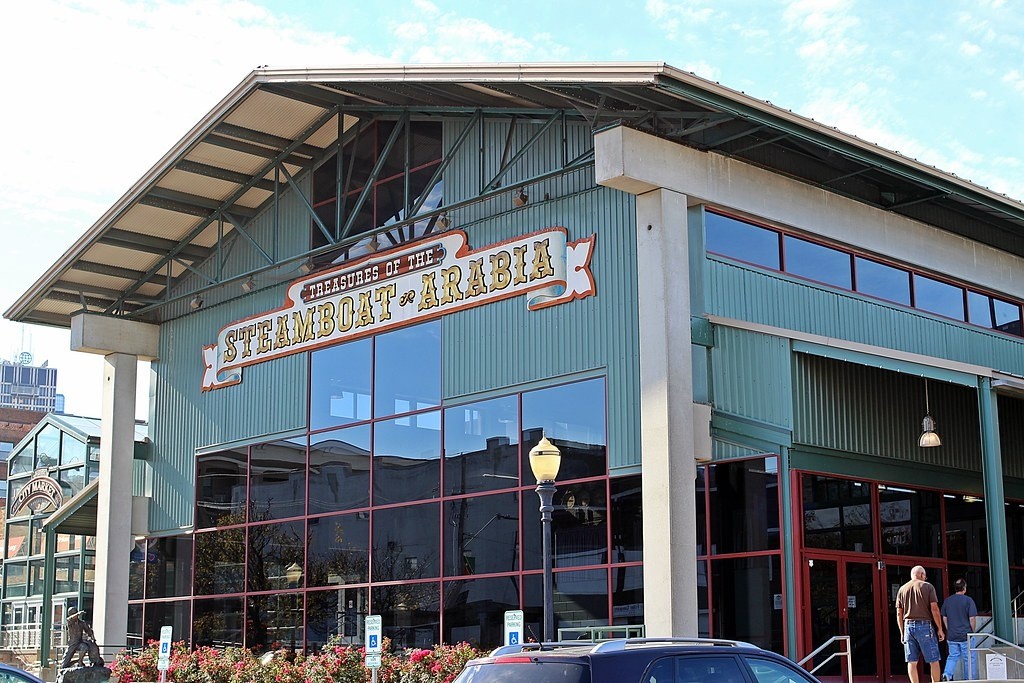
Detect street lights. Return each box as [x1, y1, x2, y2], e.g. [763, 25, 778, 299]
[528, 427, 562, 642]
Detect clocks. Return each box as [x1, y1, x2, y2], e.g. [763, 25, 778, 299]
[19, 352, 32, 364]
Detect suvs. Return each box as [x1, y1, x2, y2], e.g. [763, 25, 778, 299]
[448, 636, 822, 683]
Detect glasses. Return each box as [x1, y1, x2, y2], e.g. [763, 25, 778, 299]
[921, 574, 929, 580]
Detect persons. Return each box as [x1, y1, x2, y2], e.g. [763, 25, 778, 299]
[895, 565, 945, 683]
[61, 607, 95, 668]
[940, 579, 978, 681]
[576, 625, 605, 640]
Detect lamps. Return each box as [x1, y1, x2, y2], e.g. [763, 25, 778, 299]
[301, 257, 314, 273]
[366, 236, 380, 252]
[920, 378, 942, 448]
[242, 277, 254, 291]
[190, 295, 203, 309]
[513, 187, 527, 206]
[437, 212, 451, 229]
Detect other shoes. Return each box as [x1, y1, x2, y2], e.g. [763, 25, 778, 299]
[941, 673, 948, 682]
